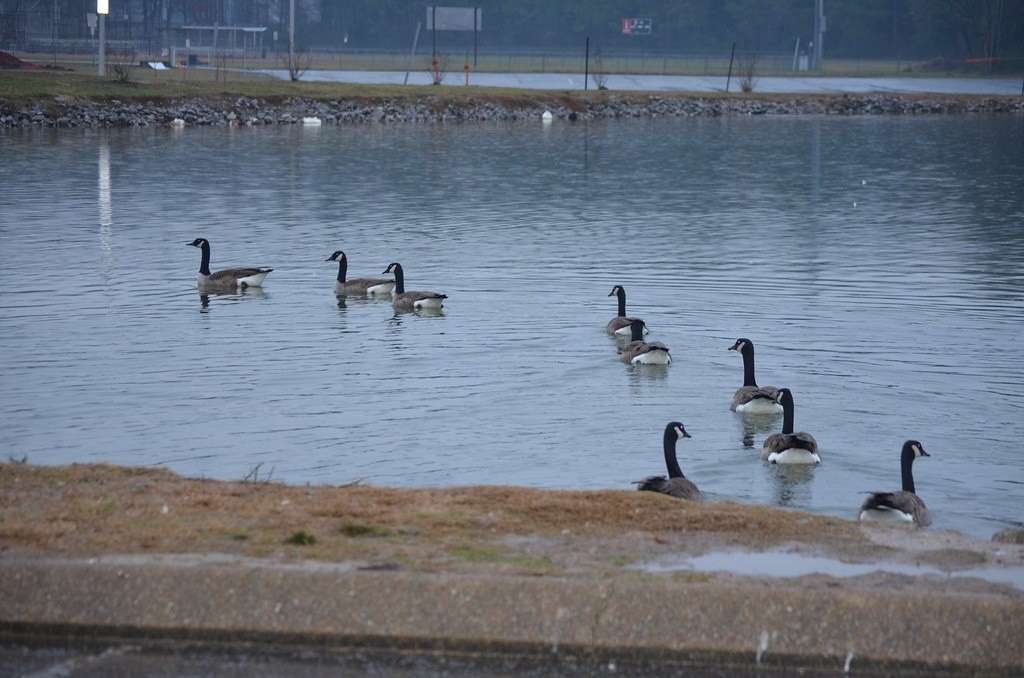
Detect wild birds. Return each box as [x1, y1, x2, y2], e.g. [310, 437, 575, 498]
[763, 388, 822, 464]
[325, 250, 396, 295]
[621, 319, 673, 365]
[186, 238, 274, 292]
[728, 338, 783, 414]
[858, 440, 932, 524]
[630, 422, 702, 500]
[606, 285, 650, 335]
[382, 263, 449, 309]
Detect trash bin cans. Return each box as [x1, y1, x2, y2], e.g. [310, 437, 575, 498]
[189, 54, 196, 65]
